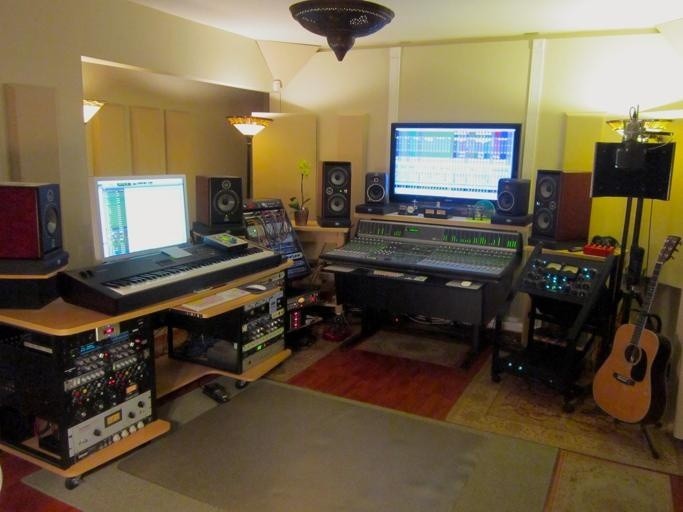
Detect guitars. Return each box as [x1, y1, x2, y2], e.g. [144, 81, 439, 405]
[591, 236, 679, 423]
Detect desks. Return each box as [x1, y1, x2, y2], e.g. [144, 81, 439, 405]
[0, 261, 292, 489]
[287, 218, 349, 315]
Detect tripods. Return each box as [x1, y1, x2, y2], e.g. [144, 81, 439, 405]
[561, 196, 662, 460]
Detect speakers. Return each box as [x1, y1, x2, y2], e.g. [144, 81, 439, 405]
[0, 181, 64, 261]
[365, 171, 387, 205]
[591, 141, 676, 201]
[196, 175, 244, 228]
[496, 178, 530, 217]
[322, 160, 351, 227]
[532, 169, 593, 242]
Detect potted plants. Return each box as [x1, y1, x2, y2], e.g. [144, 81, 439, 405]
[285, 156, 312, 226]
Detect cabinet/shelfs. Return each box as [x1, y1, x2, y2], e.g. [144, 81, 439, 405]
[319, 201, 533, 385]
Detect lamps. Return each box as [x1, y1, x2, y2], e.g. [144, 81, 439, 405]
[287, 0, 394, 62]
[223, 113, 270, 137]
[606, 119, 671, 326]
[83, 99, 104, 125]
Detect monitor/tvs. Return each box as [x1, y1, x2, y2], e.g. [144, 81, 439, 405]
[87, 174, 190, 263]
[389, 123, 521, 205]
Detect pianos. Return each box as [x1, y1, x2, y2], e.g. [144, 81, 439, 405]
[56, 237, 281, 315]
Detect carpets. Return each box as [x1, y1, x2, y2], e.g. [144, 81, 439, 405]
[444, 354, 683, 477]
[20, 379, 560, 512]
[542, 449, 676, 512]
